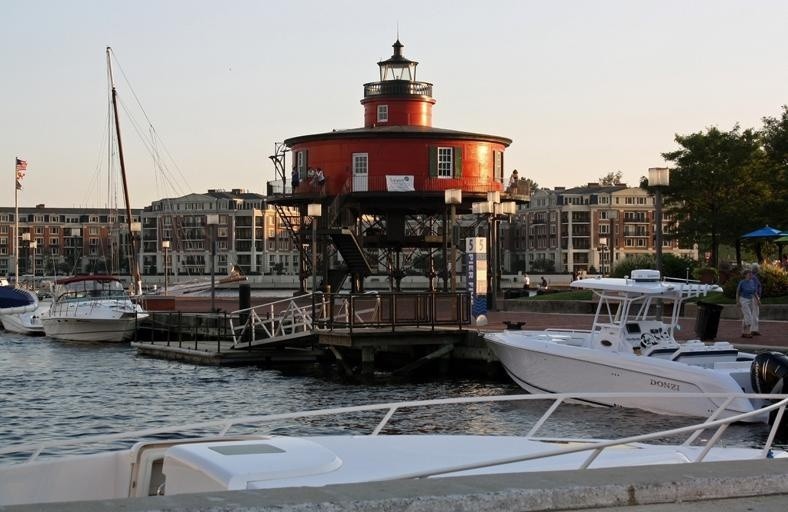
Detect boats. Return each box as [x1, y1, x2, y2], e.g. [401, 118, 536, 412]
[1, 286, 45, 336]
[40, 274, 150, 343]
[476, 267, 787, 427]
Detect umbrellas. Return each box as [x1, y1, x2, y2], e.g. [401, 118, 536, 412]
[741, 224, 788, 265]
[773, 236, 788, 242]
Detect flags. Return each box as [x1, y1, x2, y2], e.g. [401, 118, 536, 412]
[15, 158, 28, 190]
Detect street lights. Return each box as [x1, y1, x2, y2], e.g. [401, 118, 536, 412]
[647, 166, 671, 321]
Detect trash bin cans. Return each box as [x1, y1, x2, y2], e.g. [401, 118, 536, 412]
[694, 300, 724, 342]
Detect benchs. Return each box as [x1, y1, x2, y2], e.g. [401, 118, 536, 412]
[644, 341, 752, 370]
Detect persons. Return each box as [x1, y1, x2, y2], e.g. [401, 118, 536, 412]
[736, 270, 761, 338]
[521, 273, 530, 290]
[509, 169, 519, 195]
[751, 263, 762, 335]
[307, 167, 315, 178]
[291, 167, 300, 193]
[539, 277, 548, 294]
[315, 168, 326, 196]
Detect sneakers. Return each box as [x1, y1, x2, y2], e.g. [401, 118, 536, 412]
[742, 331, 762, 338]
